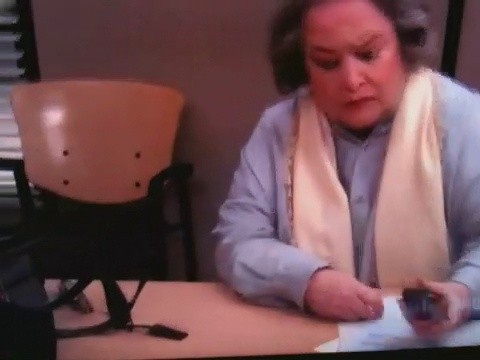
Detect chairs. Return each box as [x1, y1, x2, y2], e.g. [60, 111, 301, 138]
[0, 81, 195, 281]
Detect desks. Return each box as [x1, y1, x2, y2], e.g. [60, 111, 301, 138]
[44, 280, 480, 360]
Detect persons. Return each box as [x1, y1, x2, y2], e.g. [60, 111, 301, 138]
[214, 0, 480, 339]
